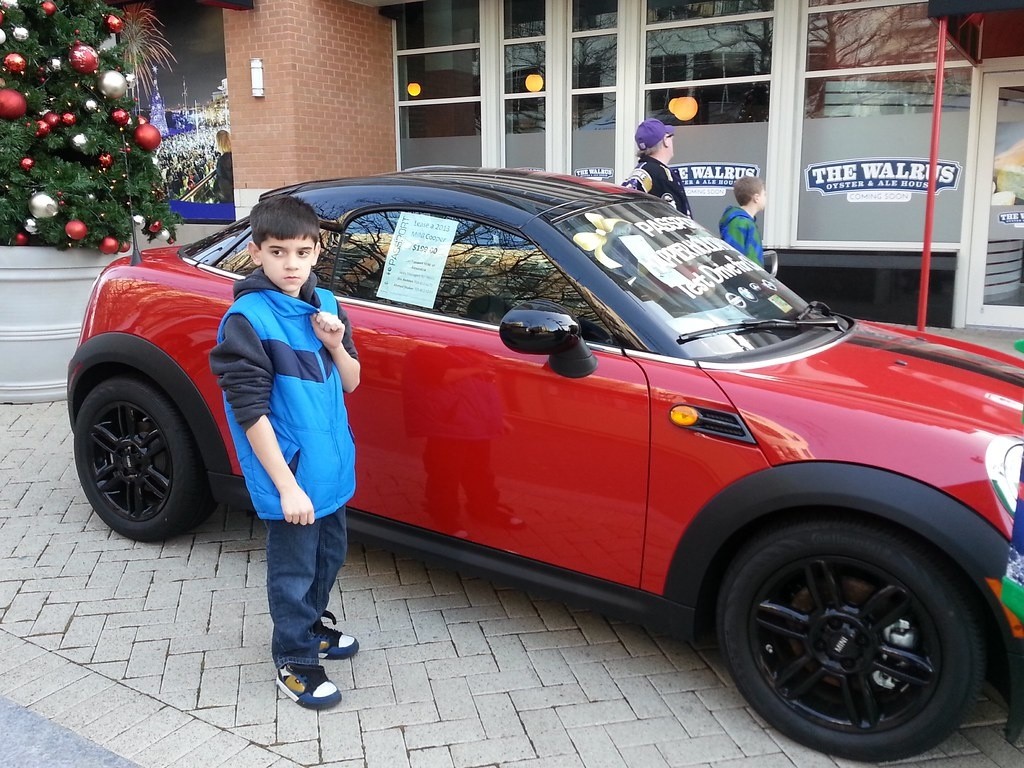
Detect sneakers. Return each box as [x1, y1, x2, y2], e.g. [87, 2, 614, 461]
[318, 625, 359, 659]
[275, 664, 343, 709]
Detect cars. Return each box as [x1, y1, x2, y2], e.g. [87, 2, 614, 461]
[66, 160, 1024, 762]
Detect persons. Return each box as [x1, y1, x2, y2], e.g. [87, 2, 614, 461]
[153, 125, 231, 199]
[210, 194, 360, 710]
[212, 130, 233, 202]
[621, 118, 693, 220]
[719, 176, 766, 270]
[424, 294, 525, 538]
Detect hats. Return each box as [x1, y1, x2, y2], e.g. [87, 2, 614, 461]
[634, 118, 674, 150]
